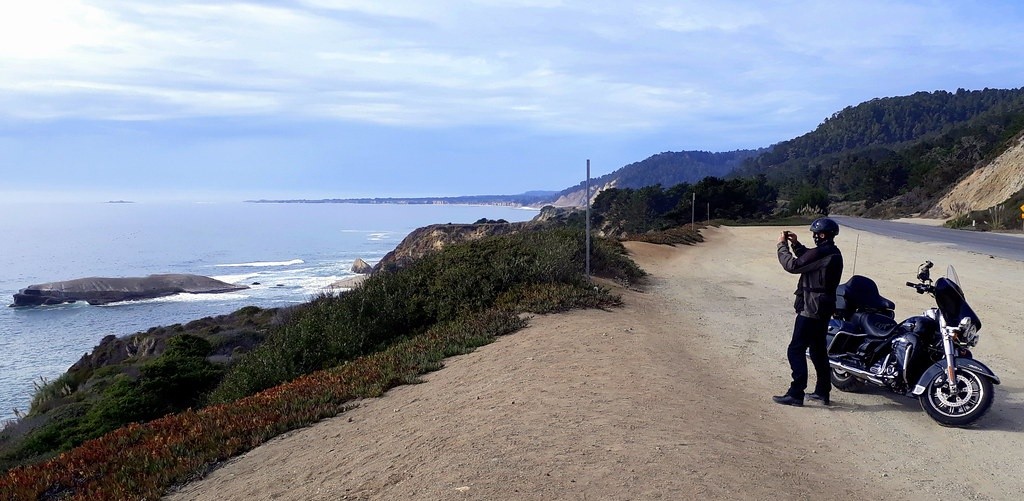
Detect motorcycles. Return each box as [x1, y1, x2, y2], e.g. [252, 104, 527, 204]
[806, 259, 1001, 427]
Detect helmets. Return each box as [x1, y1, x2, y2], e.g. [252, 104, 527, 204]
[810, 218, 839, 235]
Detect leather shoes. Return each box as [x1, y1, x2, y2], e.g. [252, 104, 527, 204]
[805, 393, 830, 404]
[773, 392, 803, 406]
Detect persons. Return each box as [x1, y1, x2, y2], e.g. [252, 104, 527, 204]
[773, 218, 843, 405]
